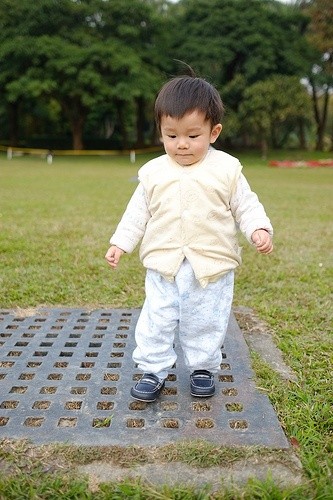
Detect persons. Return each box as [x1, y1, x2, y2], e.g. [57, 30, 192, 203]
[103, 56, 274, 403]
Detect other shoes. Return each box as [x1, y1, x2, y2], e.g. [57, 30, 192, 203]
[189, 370, 215, 397]
[130, 373, 165, 402]
